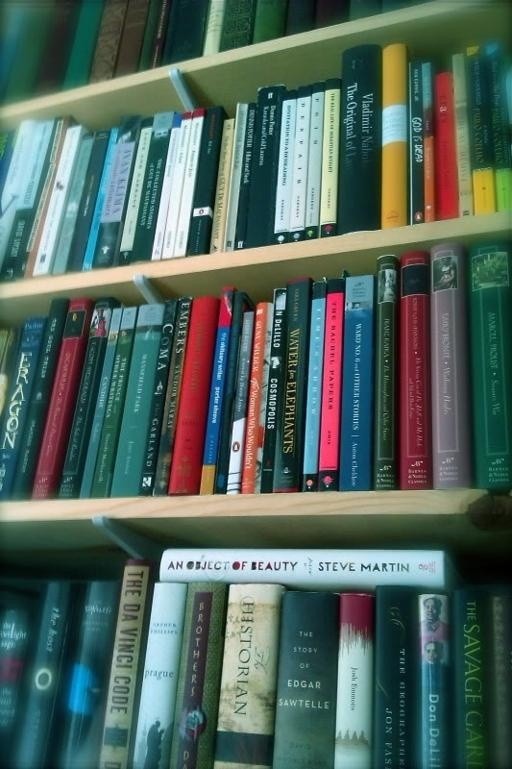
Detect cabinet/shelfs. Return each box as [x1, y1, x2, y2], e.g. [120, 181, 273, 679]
[1, 0, 512, 769]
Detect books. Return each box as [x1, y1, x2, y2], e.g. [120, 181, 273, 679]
[0, 0, 421, 103]
[0, 241, 512, 501]
[0, 546, 512, 769]
[0, 41, 512, 281]
